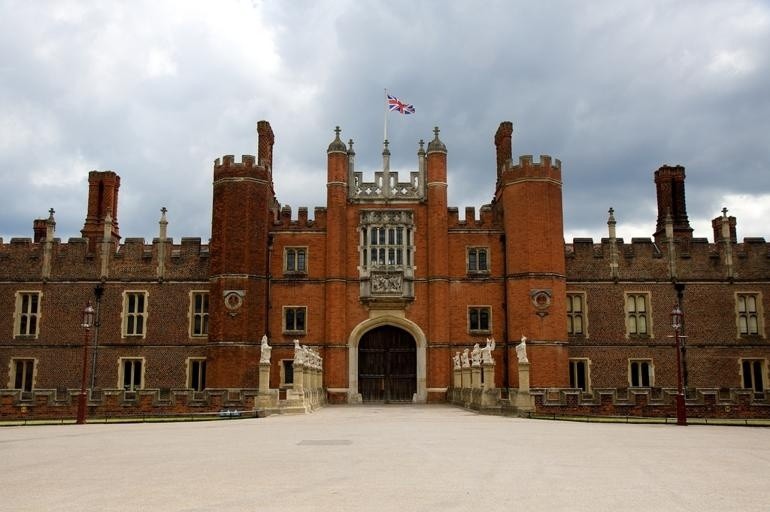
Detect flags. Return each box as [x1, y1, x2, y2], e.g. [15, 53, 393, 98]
[385, 93, 416, 116]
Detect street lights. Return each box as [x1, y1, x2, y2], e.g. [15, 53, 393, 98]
[670, 304, 688, 426]
[76, 300, 96, 424]
